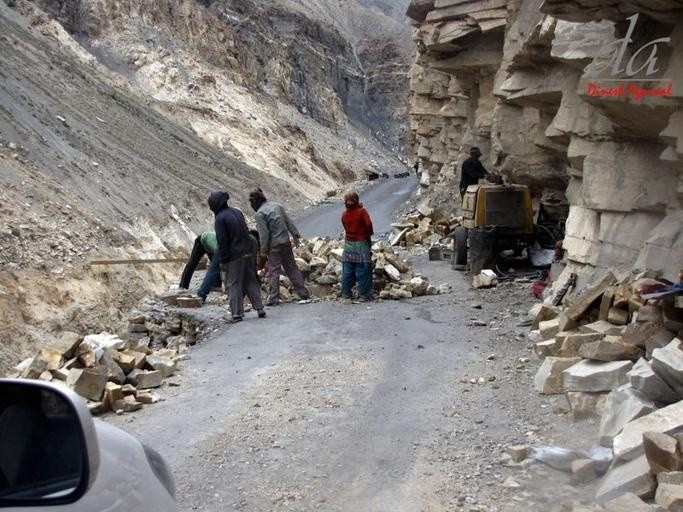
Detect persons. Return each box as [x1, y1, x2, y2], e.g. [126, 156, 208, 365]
[179, 188, 309, 320]
[459, 147, 497, 204]
[341, 190, 373, 302]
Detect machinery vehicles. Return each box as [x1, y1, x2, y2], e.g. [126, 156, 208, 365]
[451, 180, 542, 272]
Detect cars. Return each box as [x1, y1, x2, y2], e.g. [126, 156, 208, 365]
[0, 373, 181, 511]
